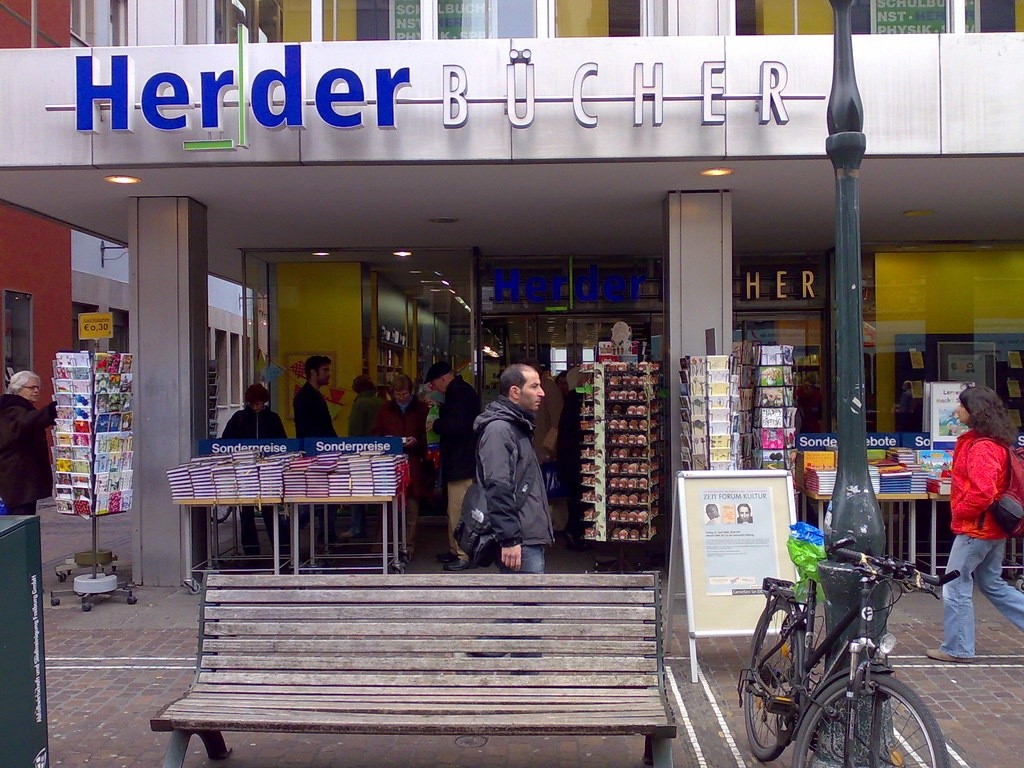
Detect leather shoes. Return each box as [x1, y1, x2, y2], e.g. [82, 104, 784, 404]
[434, 551, 457, 562]
[443, 558, 471, 571]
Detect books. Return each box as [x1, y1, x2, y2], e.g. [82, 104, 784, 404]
[50, 351, 132, 519]
[795, 448, 953, 495]
[165, 450, 408, 500]
[678, 345, 797, 470]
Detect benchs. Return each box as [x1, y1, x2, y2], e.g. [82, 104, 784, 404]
[147, 562, 680, 768]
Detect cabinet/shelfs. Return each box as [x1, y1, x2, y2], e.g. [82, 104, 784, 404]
[573, 363, 662, 542]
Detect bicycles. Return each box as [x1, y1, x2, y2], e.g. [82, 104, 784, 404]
[738, 536, 960, 768]
[210, 505, 232, 524]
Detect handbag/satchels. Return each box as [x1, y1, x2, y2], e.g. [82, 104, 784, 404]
[540, 460, 566, 498]
[454, 483, 500, 568]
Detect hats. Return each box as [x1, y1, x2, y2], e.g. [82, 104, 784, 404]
[424, 361, 451, 384]
[245, 383, 269, 401]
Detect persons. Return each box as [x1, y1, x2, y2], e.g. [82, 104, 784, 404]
[221, 384, 288, 563]
[0, 371, 57, 515]
[796, 374, 827, 433]
[737, 503, 753, 523]
[341, 361, 479, 571]
[926, 386, 1024, 663]
[458, 364, 556, 676]
[706, 504, 720, 524]
[899, 381, 914, 432]
[967, 363, 974, 373]
[533, 363, 592, 552]
[283, 356, 348, 544]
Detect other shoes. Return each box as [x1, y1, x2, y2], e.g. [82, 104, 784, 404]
[566, 532, 592, 550]
[926, 649, 973, 662]
[340, 530, 367, 538]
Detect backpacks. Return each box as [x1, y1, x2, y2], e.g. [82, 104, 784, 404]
[967, 437, 1024, 540]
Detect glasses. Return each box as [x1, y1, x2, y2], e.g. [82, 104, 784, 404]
[23, 385, 39, 390]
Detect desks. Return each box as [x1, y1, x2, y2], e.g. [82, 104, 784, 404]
[284, 495, 400, 576]
[799, 487, 931, 568]
[170, 495, 294, 579]
[909, 491, 1024, 585]
